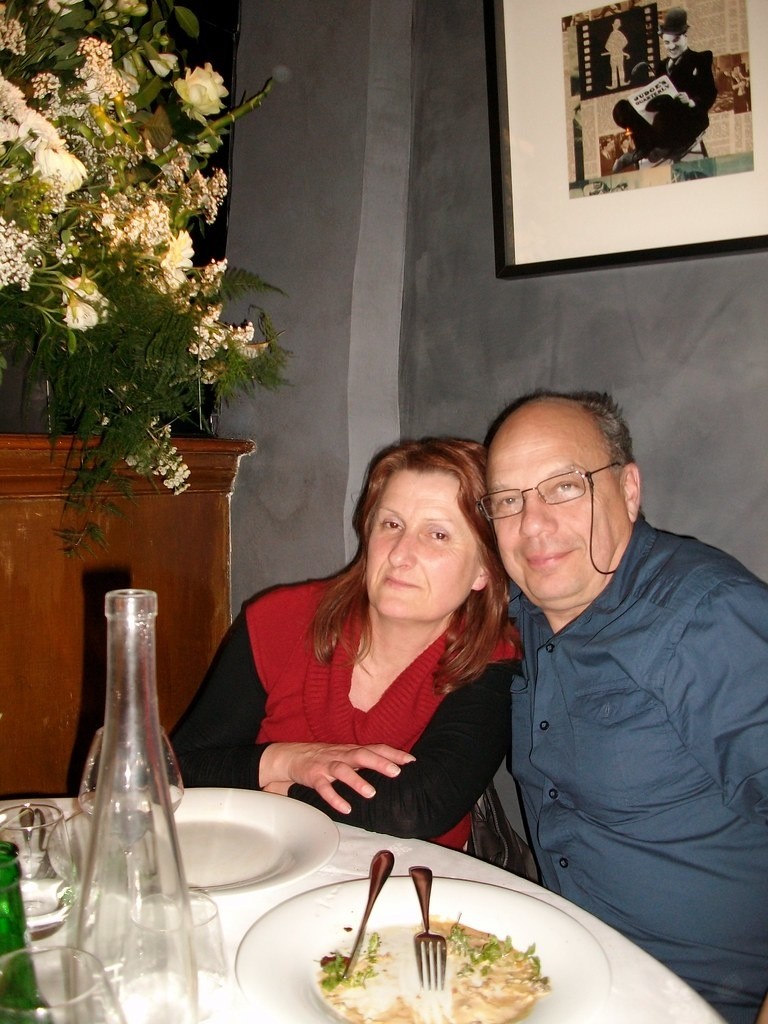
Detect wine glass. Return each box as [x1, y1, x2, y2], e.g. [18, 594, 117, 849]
[78, 724, 217, 927]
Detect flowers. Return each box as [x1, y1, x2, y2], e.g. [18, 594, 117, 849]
[0, 0, 296, 561]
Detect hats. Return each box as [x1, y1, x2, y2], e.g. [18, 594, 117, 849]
[660, 9, 688, 35]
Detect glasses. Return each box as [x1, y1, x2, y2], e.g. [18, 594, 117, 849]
[479, 463, 620, 521]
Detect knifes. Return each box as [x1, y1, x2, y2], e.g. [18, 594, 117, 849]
[343, 851, 395, 981]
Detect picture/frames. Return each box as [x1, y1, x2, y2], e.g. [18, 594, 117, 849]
[483, 0, 768, 281]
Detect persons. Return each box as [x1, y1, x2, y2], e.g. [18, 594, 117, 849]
[477, 388, 768, 1024]
[612, 8, 716, 173]
[169, 438, 521, 850]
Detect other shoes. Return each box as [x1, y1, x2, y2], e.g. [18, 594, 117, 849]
[632, 146, 651, 162]
[650, 139, 671, 163]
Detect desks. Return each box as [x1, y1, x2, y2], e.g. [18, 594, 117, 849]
[0, 797, 730, 1024]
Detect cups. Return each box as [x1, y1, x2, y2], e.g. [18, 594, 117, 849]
[1, 804, 74, 940]
[123, 895, 225, 1019]
[2, 945, 117, 1023]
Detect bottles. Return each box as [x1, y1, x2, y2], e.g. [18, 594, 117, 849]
[0, 840, 40, 1021]
[76, 591, 200, 1021]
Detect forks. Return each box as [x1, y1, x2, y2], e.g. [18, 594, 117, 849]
[410, 866, 446, 989]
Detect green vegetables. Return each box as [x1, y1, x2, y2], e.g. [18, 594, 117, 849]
[318, 910, 542, 992]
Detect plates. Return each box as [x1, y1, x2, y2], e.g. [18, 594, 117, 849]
[235, 874, 609, 1023]
[50, 787, 338, 903]
